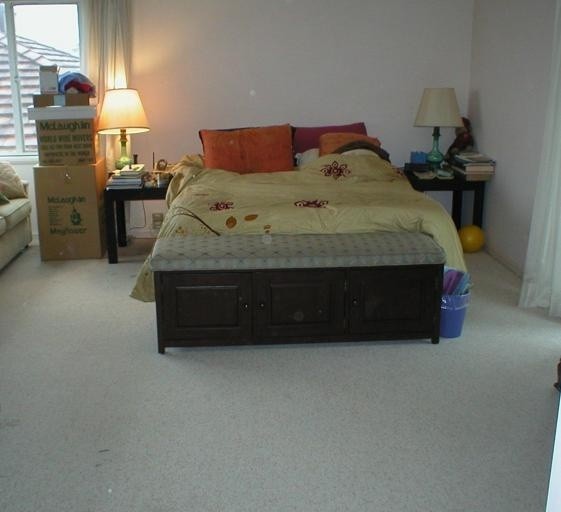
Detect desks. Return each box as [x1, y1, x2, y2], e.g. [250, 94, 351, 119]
[405, 163, 485, 234]
[106, 170, 170, 266]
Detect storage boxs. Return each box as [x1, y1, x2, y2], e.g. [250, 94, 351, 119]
[31, 160, 107, 262]
[26, 93, 102, 166]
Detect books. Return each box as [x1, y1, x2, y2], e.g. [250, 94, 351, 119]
[105, 164, 149, 190]
[451, 152, 496, 181]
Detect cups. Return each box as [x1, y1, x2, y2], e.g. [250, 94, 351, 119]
[157, 174, 168, 189]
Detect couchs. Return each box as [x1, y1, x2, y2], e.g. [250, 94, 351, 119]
[0, 161, 31, 274]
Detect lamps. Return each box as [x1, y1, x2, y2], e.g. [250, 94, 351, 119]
[98, 87, 148, 170]
[413, 87, 466, 163]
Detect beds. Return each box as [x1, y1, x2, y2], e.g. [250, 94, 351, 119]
[128, 122, 453, 357]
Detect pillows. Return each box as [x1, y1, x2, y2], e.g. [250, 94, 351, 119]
[295, 146, 319, 169]
[199, 122, 295, 173]
[1, 162, 28, 199]
[319, 131, 382, 157]
[292, 122, 368, 150]
